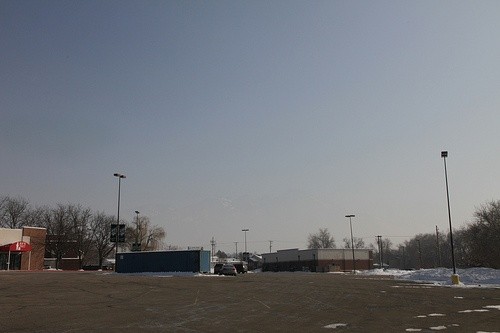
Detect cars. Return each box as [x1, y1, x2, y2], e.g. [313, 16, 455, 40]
[214, 263, 224, 274]
[219, 263, 238, 277]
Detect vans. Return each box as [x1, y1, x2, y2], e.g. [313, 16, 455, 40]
[234, 261, 248, 274]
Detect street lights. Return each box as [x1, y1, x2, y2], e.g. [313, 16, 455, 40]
[345, 214, 356, 274]
[377, 235, 384, 267]
[242, 229, 249, 261]
[440, 150, 457, 273]
[416, 239, 423, 267]
[113, 172, 126, 253]
[134, 210, 140, 243]
[435, 224, 442, 267]
